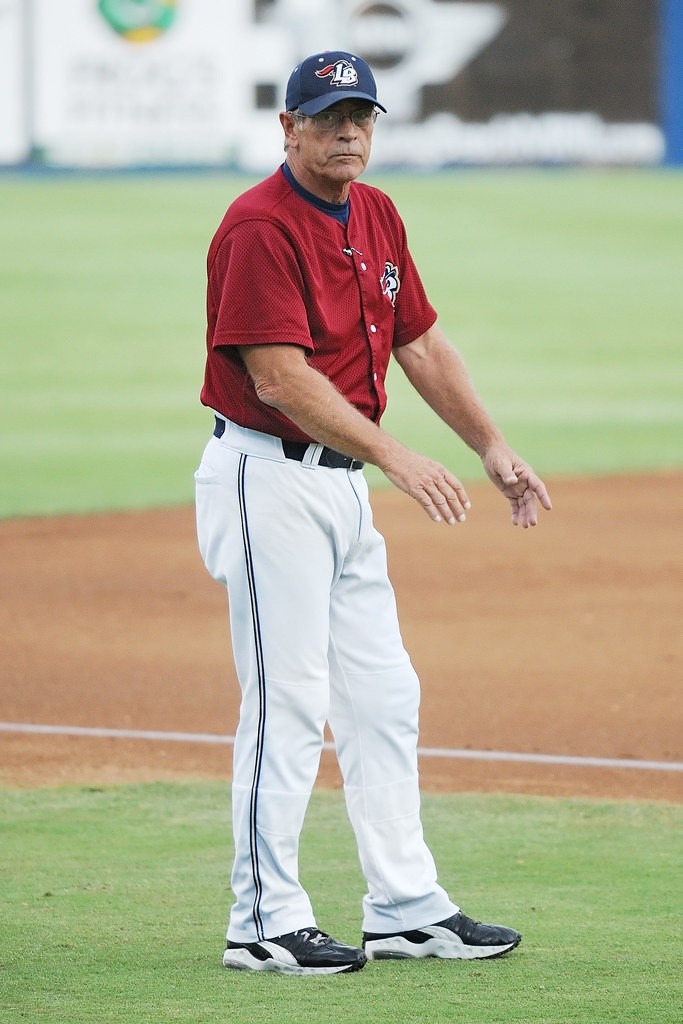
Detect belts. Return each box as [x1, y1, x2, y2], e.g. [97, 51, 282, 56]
[213, 413, 365, 470]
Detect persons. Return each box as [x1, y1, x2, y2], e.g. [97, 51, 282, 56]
[193, 52, 555, 975]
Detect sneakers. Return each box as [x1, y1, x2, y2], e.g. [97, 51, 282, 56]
[361, 908, 523, 960]
[222, 927, 368, 975]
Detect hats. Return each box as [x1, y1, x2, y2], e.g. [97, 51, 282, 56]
[285, 51, 387, 117]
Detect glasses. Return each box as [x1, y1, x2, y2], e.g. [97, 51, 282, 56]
[289, 108, 380, 130]
